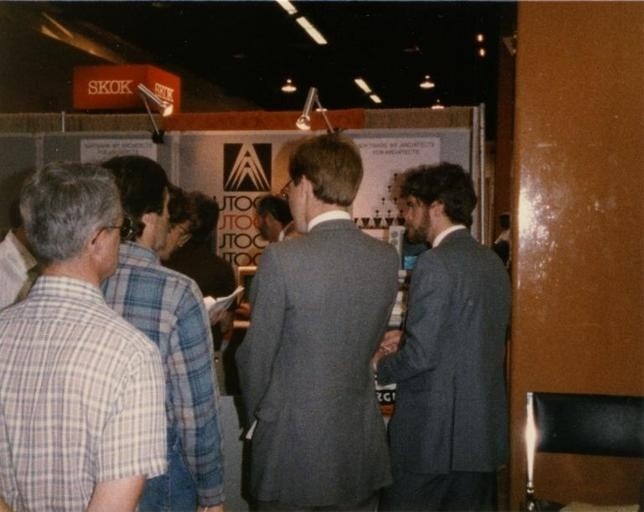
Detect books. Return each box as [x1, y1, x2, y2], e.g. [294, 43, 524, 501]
[203, 285, 244, 327]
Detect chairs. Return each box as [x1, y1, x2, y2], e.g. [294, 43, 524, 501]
[517, 392, 644, 512]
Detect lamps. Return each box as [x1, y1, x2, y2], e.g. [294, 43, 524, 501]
[136, 82, 174, 145]
[295, 87, 336, 137]
[419, 75, 435, 89]
[281, 78, 297, 93]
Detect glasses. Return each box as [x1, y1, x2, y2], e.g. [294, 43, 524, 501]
[90, 216, 136, 244]
[279, 178, 297, 200]
[167, 223, 193, 248]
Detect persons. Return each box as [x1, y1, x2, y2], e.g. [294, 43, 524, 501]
[0, 160, 168, 512]
[96, 154, 226, 512]
[233, 130, 398, 508]
[371, 163, 513, 510]
[233, 192, 303, 320]
[155, 186, 234, 347]
[0, 198, 40, 309]
